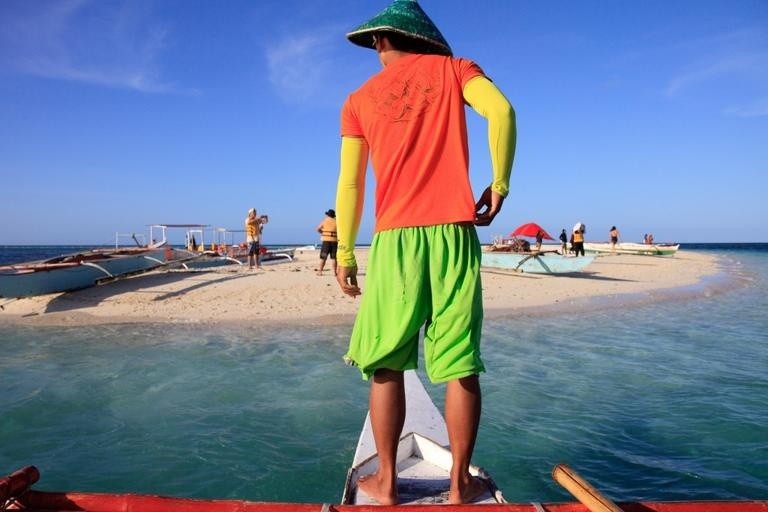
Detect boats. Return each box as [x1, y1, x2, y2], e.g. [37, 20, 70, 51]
[0, 223, 294, 299]
[0, 464, 767, 512]
[482, 249, 598, 275]
[491, 240, 682, 257]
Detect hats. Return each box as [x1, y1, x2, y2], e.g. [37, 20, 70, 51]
[345, 0, 454, 58]
[249, 208, 256, 214]
[325, 209, 336, 218]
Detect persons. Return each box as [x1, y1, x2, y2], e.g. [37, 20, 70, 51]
[245, 208, 263, 271]
[559, 229, 568, 255]
[642, 234, 654, 245]
[573, 224, 585, 257]
[570, 234, 576, 254]
[331, 1, 517, 507]
[315, 209, 337, 277]
[535, 230, 544, 251]
[609, 226, 619, 248]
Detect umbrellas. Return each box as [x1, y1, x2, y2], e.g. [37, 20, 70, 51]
[508, 223, 556, 251]
